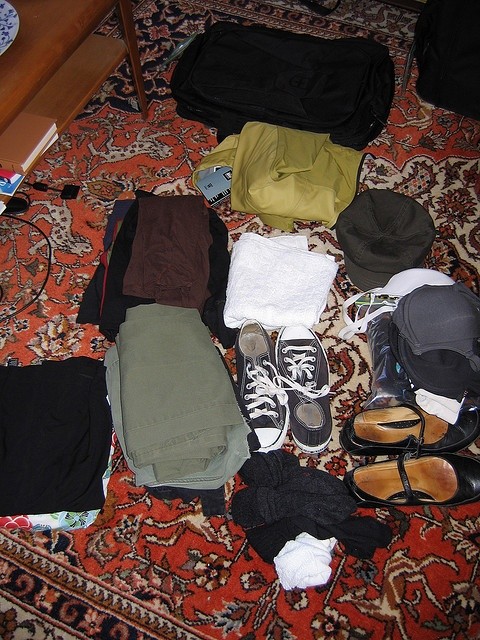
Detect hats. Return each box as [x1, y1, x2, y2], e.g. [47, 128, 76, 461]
[335, 189, 436, 291]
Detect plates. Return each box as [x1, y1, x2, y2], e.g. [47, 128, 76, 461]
[0, 1, 20, 56]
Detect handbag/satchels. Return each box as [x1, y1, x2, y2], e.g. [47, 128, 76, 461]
[412, 0, 479, 120]
[169, 20, 395, 152]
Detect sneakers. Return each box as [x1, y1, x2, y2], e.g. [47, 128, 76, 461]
[275, 324, 336, 454]
[234, 320, 289, 453]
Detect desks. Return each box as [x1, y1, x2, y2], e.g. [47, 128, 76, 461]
[0, 0, 149, 214]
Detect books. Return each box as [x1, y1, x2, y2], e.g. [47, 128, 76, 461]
[0, 111, 61, 197]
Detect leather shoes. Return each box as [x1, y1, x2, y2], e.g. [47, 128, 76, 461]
[343, 451, 480, 508]
[339, 403, 480, 456]
